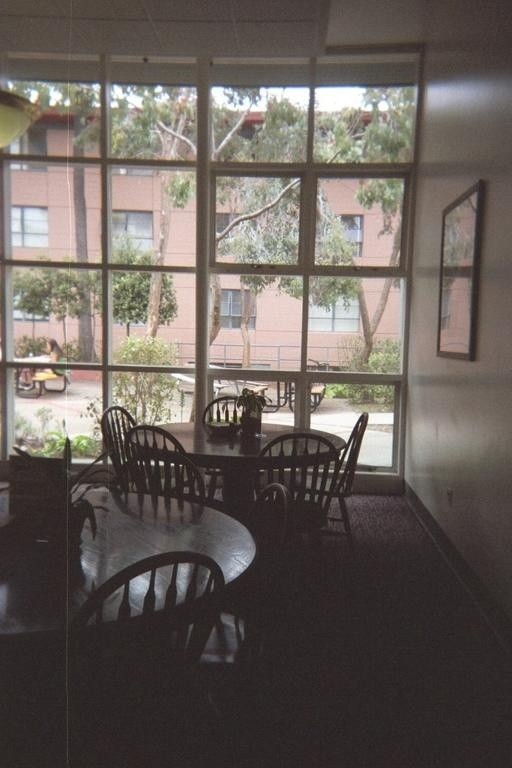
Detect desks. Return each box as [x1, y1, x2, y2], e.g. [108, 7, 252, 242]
[0, 487, 258, 768]
[126, 420, 346, 540]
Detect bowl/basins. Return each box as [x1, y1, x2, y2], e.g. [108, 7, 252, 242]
[208, 422, 240, 435]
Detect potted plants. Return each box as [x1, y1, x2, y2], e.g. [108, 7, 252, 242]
[236, 389, 264, 426]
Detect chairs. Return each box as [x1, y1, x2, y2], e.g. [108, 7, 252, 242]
[122, 427, 194, 497]
[101, 409, 142, 487]
[286, 413, 368, 541]
[203, 395, 249, 424]
[54, 550, 222, 760]
[249, 434, 339, 586]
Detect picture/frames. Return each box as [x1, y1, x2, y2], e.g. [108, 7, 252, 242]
[435, 179, 489, 361]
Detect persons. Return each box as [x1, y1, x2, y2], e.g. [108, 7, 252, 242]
[35, 338, 65, 394]
